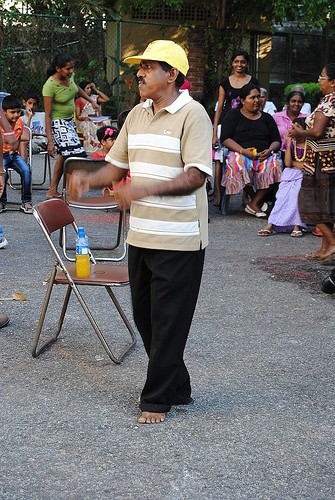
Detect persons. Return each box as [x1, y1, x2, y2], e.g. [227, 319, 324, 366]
[70, 40, 212, 424]
[0, 95, 32, 250]
[259, 117, 309, 237]
[271, 91, 310, 167]
[259, 88, 276, 115]
[288, 64, 335, 260]
[206, 93, 226, 201]
[211, 50, 259, 206]
[89, 126, 120, 159]
[42, 53, 100, 202]
[220, 83, 283, 217]
[20, 93, 59, 160]
[75, 80, 110, 150]
[284, 85, 312, 116]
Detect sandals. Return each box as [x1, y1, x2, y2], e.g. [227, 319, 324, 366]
[0, 202, 8, 213]
[20, 202, 33, 213]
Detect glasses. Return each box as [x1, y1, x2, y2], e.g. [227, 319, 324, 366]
[319, 75, 327, 80]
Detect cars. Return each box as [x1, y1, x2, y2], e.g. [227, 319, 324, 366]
[0, 92, 24, 116]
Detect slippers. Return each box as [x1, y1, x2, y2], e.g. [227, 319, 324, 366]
[291, 229, 303, 236]
[258, 228, 277, 235]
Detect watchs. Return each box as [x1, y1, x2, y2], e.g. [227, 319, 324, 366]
[268, 148, 273, 155]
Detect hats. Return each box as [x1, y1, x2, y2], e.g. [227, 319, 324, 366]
[124, 39, 189, 77]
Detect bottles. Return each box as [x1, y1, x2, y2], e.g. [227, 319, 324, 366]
[75, 233, 90, 277]
[76, 227, 88, 256]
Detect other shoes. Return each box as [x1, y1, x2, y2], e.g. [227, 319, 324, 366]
[244, 202, 273, 219]
[311, 230, 323, 236]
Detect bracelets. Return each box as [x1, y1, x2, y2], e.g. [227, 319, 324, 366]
[240, 147, 244, 155]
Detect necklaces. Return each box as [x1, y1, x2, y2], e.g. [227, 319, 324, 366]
[293, 137, 308, 162]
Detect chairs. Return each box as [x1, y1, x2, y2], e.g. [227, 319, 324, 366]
[0, 126, 35, 212]
[32, 198, 136, 364]
[59, 158, 128, 261]
[8, 133, 53, 190]
